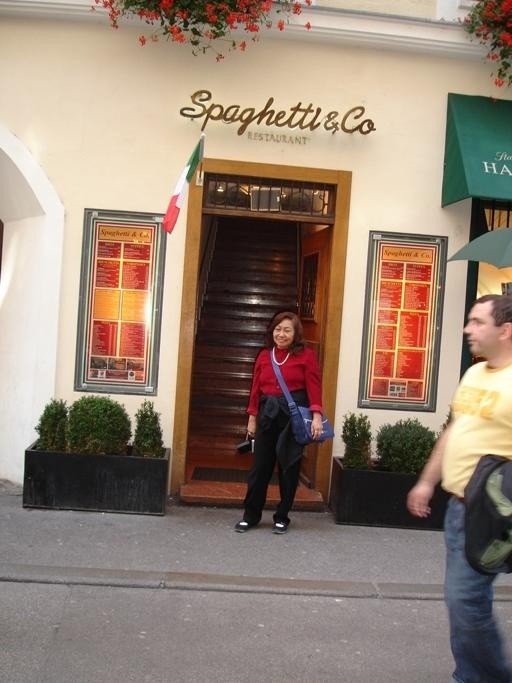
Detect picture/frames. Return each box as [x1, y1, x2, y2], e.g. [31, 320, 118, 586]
[358, 230, 448, 412]
[74, 207, 165, 396]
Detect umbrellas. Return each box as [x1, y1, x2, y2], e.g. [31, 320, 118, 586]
[445, 227, 512, 269]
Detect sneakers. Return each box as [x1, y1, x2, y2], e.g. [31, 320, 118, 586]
[234, 520, 249, 533]
[271, 519, 288, 534]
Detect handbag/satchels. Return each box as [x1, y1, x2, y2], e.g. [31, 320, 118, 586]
[291, 403, 335, 445]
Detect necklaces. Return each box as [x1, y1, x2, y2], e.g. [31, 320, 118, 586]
[272, 346, 290, 365]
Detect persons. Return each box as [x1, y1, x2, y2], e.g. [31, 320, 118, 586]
[407, 294, 512, 683]
[235, 311, 335, 534]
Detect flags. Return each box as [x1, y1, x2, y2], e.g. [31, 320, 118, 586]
[162, 134, 204, 234]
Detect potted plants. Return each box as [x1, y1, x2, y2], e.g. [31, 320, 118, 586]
[22, 395, 172, 516]
[328, 412, 444, 534]
